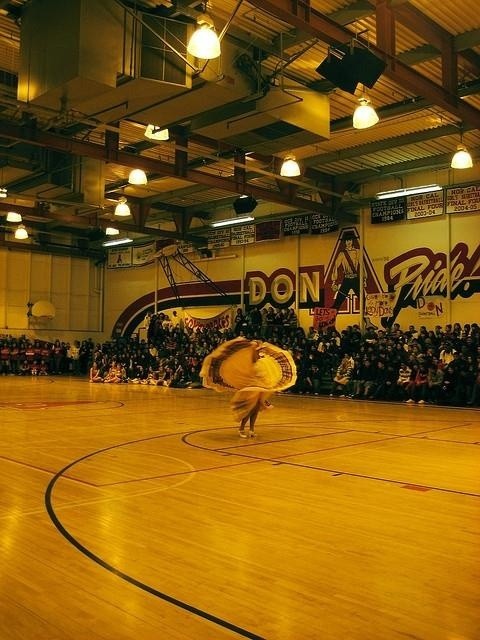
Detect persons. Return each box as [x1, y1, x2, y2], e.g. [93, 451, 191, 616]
[330, 231, 377, 329]
[198, 335, 297, 438]
[299, 323, 478, 407]
[0, 333, 94, 376]
[90, 308, 306, 395]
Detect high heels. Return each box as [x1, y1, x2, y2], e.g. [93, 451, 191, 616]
[249, 431, 256, 437]
[238, 430, 248, 438]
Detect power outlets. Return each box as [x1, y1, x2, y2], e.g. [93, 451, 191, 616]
[353, 85, 381, 132]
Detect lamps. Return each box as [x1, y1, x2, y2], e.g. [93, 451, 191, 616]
[6, 123, 302, 242]
[100, 238, 133, 249]
[186, 15, 222, 61]
[450, 123, 474, 170]
[375, 183, 443, 201]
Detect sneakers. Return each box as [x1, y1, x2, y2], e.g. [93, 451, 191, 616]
[406, 399, 414, 403]
[418, 400, 424, 403]
